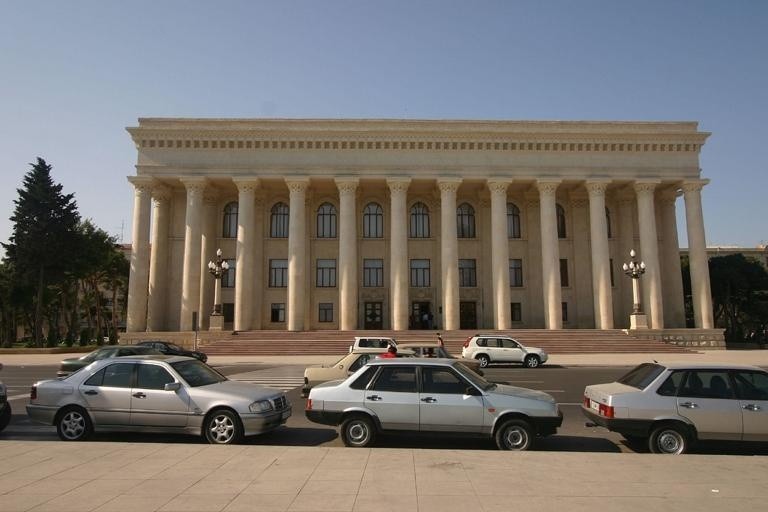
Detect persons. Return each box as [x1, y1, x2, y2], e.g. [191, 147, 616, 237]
[423, 312, 428, 329]
[375, 344, 398, 358]
[427, 311, 434, 330]
[436, 332, 444, 349]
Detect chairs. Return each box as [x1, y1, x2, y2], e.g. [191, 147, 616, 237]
[687, 374, 727, 394]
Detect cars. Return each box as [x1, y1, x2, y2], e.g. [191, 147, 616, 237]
[59, 341, 211, 375]
[305, 356, 561, 450]
[305, 331, 482, 399]
[462, 335, 549, 369]
[25, 354, 292, 442]
[582, 362, 768, 453]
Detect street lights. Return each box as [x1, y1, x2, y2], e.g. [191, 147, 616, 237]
[624, 247, 647, 315]
[207, 249, 230, 316]
